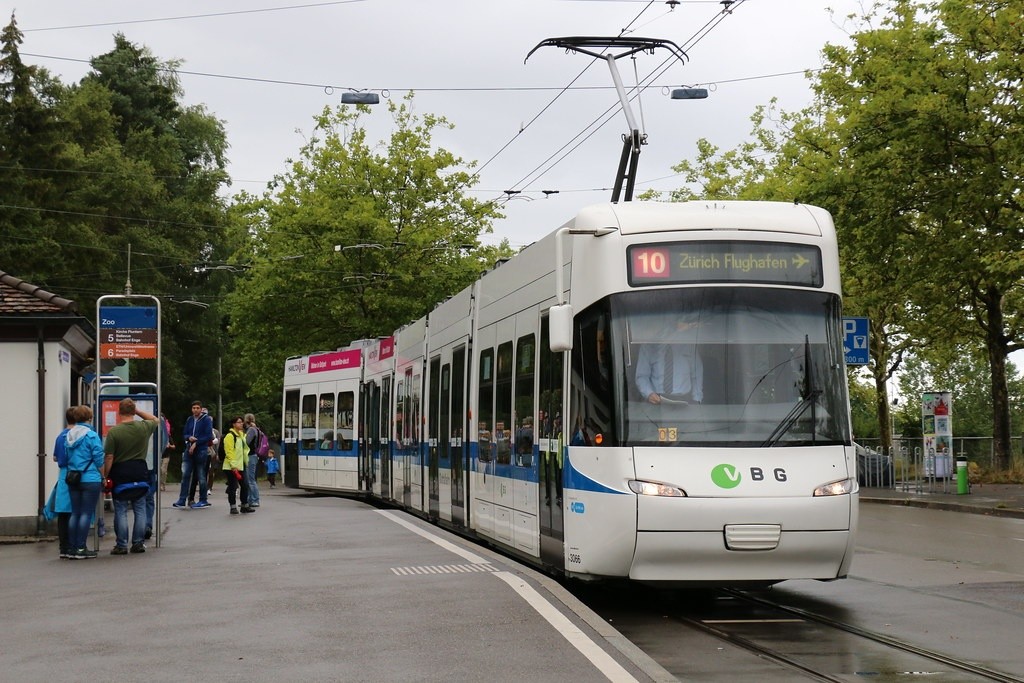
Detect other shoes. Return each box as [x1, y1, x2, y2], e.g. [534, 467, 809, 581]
[145, 527, 152, 539]
[269, 485, 275, 489]
[190, 500, 211, 508]
[59, 547, 76, 558]
[130, 540, 146, 553]
[240, 507, 256, 512]
[110, 546, 128, 554]
[207, 488, 211, 495]
[173, 501, 186, 509]
[188, 499, 196, 506]
[75, 547, 97, 558]
[161, 483, 165, 491]
[238, 502, 260, 507]
[230, 508, 239, 515]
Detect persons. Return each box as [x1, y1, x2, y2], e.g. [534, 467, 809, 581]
[157, 401, 260, 514]
[634, 344, 705, 404]
[103, 399, 159, 555]
[320, 430, 345, 450]
[262, 449, 280, 489]
[451, 410, 585, 509]
[136, 392, 168, 540]
[42, 407, 76, 558]
[63, 405, 106, 559]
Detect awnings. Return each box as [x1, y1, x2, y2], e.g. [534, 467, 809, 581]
[627, 299, 828, 345]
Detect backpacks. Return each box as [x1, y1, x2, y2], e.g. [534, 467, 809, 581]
[246, 426, 269, 458]
[218, 431, 239, 461]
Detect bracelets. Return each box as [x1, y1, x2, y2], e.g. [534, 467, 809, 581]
[102, 476, 106, 480]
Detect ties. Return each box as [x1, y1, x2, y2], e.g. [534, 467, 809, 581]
[664, 344, 673, 394]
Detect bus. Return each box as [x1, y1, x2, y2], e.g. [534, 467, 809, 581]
[279, 201, 860, 583]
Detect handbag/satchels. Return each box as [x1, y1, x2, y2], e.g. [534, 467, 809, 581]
[65, 470, 81, 486]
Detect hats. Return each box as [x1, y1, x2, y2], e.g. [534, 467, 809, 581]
[544, 412, 548, 419]
[201, 408, 208, 414]
[555, 412, 562, 418]
[522, 418, 532, 424]
